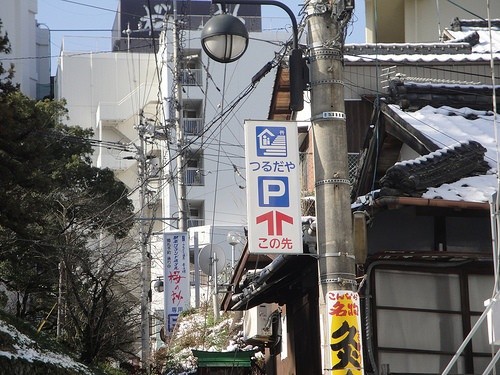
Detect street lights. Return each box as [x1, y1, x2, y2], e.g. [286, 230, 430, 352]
[201, 0, 371, 374]
